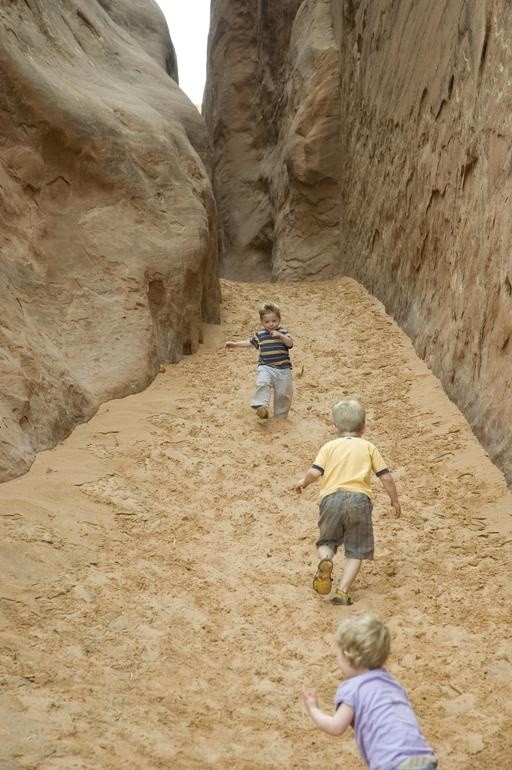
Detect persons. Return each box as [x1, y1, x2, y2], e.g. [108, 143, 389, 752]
[294, 399, 402, 606]
[222, 303, 294, 420]
[301, 612, 439, 769]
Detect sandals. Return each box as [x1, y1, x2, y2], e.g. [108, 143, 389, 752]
[313, 559, 333, 594]
[333, 589, 354, 604]
